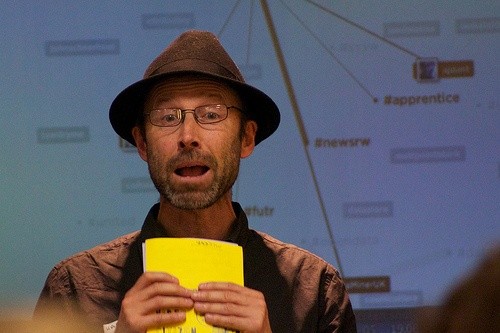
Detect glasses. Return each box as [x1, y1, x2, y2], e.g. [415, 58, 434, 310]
[144, 104, 242, 127]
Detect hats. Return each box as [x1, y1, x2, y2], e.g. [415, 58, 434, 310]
[108, 29, 281, 147]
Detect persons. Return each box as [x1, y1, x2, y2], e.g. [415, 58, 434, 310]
[32, 31, 360, 333]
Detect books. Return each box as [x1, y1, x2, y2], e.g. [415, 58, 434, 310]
[142, 237, 245, 333]
